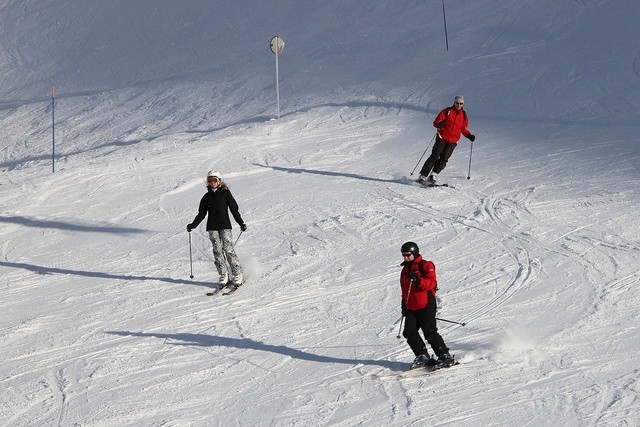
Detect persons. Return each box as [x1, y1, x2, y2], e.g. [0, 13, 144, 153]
[416, 95, 476, 185]
[185, 169, 248, 287]
[398, 241, 455, 373]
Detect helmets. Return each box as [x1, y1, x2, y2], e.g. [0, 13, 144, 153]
[455, 96, 464, 104]
[401, 242, 419, 256]
[207, 170, 221, 181]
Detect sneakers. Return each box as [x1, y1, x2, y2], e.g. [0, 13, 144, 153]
[439, 352, 452, 364]
[429, 172, 437, 183]
[219, 273, 229, 285]
[419, 175, 426, 182]
[413, 354, 430, 365]
[232, 274, 242, 284]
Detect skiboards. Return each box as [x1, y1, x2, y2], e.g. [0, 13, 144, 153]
[406, 358, 459, 371]
[416, 181, 449, 187]
[207, 280, 245, 296]
